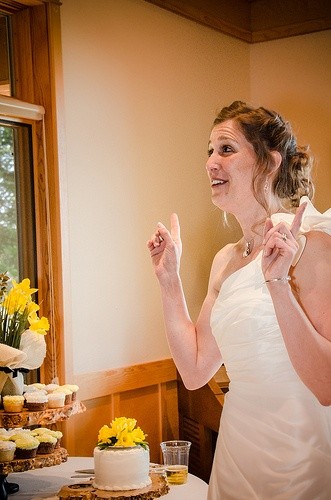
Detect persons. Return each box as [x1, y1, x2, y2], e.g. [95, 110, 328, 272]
[147, 99, 331, 500]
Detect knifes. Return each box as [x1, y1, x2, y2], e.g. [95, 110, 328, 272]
[70, 474, 94, 478]
[74, 469, 93, 474]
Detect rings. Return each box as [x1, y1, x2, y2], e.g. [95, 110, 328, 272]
[282, 234, 286, 242]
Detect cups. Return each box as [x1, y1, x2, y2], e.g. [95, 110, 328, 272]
[159, 439, 192, 486]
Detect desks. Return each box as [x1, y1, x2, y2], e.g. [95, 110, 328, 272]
[6, 456, 209, 500]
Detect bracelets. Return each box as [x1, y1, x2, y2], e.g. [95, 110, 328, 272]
[265, 276, 291, 283]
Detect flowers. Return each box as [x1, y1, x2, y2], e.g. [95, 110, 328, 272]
[0, 271, 50, 350]
[96, 416, 149, 451]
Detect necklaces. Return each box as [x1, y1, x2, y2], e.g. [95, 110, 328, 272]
[243, 226, 266, 256]
